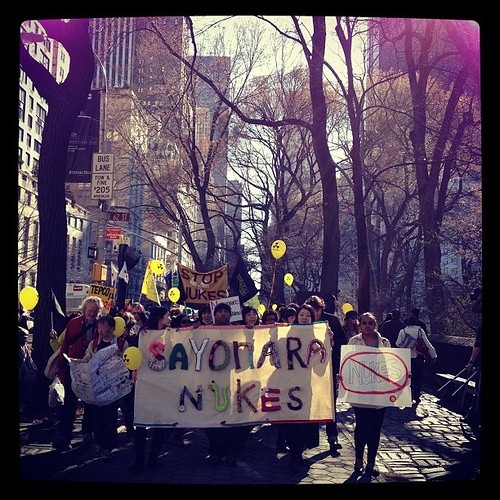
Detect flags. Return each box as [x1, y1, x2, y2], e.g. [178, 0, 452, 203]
[110, 262, 118, 286]
[119, 261, 129, 284]
[141, 261, 161, 307]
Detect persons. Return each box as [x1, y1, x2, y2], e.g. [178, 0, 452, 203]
[460, 325, 481, 423]
[336, 312, 392, 476]
[18, 296, 437, 477]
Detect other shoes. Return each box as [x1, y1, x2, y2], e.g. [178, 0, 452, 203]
[55, 432, 122, 458]
[329, 442, 341, 452]
[353, 466, 364, 474]
[287, 452, 309, 469]
[365, 466, 379, 476]
[206, 453, 229, 465]
[135, 459, 160, 468]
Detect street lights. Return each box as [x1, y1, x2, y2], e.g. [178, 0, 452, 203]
[20, 29, 111, 307]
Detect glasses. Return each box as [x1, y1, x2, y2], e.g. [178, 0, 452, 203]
[180, 321, 191, 324]
[350, 316, 358, 320]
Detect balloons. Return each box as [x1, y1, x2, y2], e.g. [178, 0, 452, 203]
[272, 303, 277, 311]
[150, 259, 166, 275]
[123, 346, 142, 371]
[113, 317, 126, 337]
[18, 285, 39, 311]
[257, 304, 265, 316]
[168, 287, 180, 303]
[284, 273, 294, 286]
[271, 239, 287, 259]
[342, 303, 353, 315]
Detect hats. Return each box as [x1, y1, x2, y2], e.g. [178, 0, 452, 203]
[343, 310, 359, 321]
